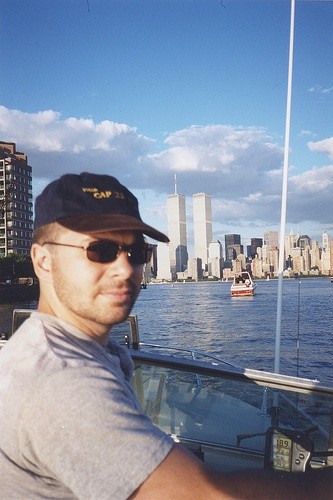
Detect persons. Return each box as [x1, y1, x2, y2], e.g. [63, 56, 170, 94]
[236, 275, 251, 286]
[1, 171, 333, 500]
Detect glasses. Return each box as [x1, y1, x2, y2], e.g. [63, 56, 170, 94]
[38, 240, 154, 266]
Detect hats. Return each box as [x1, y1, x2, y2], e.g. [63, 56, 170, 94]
[33, 172, 171, 245]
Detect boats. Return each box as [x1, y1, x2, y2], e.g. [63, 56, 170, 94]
[231, 272, 257, 296]
